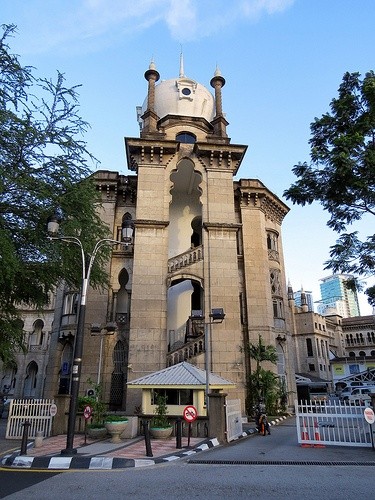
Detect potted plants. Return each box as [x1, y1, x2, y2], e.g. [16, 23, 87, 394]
[79, 377, 106, 438]
[148, 392, 173, 438]
[105, 415, 129, 442]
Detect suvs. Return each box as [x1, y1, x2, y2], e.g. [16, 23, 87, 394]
[339, 386, 367, 406]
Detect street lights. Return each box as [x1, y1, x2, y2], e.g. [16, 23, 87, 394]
[90, 321, 118, 403]
[46, 221, 134, 460]
[189, 307, 226, 416]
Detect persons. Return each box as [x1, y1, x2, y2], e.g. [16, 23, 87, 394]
[255, 401, 271, 435]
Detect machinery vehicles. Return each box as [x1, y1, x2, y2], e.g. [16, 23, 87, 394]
[295, 374, 328, 412]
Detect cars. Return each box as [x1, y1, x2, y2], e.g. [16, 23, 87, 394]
[348, 388, 373, 405]
